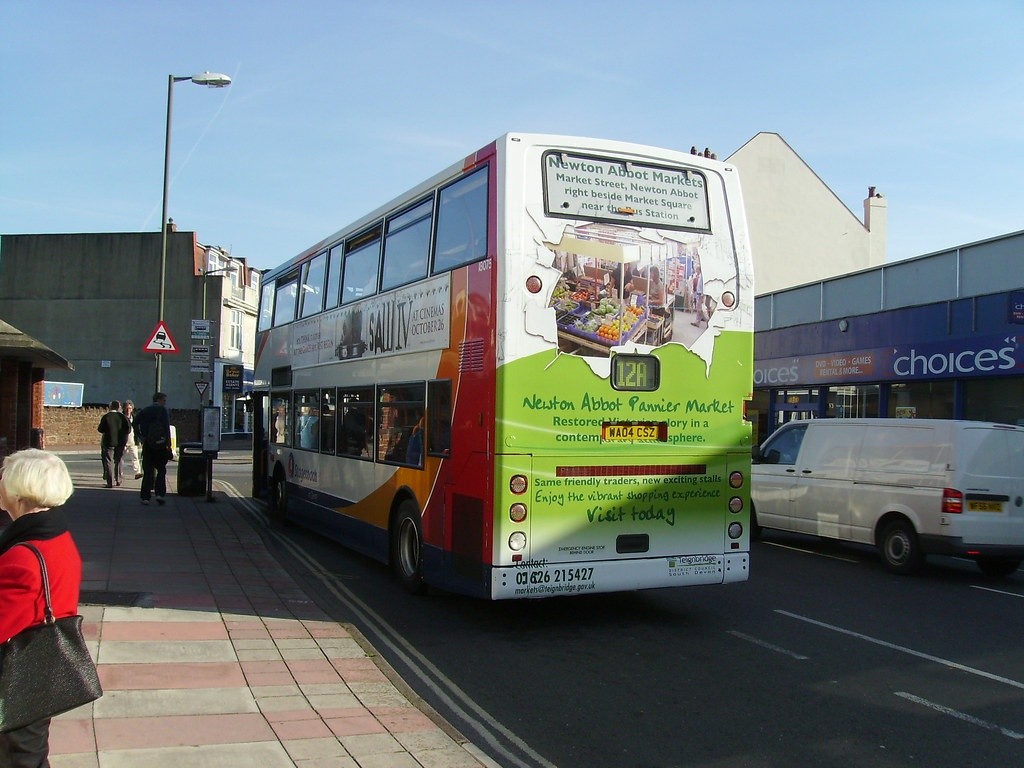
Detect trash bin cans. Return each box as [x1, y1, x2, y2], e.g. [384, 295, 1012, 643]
[33, 429, 44, 450]
[177, 442, 208, 498]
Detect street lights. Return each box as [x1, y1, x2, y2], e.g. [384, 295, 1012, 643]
[154, 71, 232, 393]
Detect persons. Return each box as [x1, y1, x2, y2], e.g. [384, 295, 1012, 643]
[0, 448, 82, 768]
[99, 400, 143, 488]
[608, 261, 713, 331]
[132, 393, 173, 505]
[275, 408, 370, 457]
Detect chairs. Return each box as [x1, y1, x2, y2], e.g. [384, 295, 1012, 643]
[406, 415, 448, 465]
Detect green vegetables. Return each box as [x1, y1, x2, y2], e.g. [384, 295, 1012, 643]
[575, 310, 603, 333]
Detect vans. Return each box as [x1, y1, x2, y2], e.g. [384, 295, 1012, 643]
[747, 418, 1024, 581]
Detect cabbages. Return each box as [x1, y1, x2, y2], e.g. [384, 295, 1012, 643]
[598, 299, 618, 315]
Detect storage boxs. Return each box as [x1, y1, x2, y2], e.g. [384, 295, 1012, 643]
[553, 300, 647, 346]
[647, 314, 664, 329]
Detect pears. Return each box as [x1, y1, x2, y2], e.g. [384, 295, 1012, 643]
[551, 286, 566, 298]
[611, 319, 632, 332]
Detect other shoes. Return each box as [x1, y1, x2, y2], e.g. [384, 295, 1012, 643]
[116, 482, 120, 486]
[140, 498, 149, 505]
[156, 496, 166, 505]
[135, 473, 143, 479]
[103, 484, 112, 488]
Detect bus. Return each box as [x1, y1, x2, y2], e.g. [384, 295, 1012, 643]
[245, 131, 756, 605]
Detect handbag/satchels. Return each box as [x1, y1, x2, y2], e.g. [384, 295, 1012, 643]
[0, 543, 103, 734]
[143, 441, 168, 468]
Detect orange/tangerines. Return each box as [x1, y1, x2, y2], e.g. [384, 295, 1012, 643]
[626, 305, 643, 316]
[598, 325, 619, 340]
